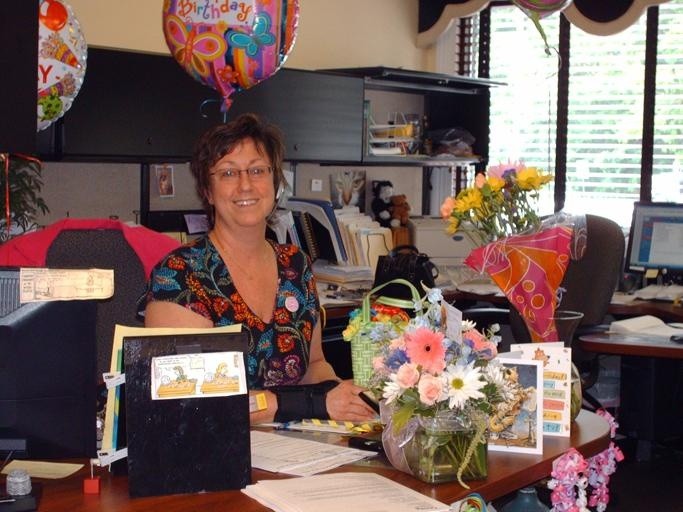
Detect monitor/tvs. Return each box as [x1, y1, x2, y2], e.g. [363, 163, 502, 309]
[0, 266, 98, 510]
[624, 201, 683, 301]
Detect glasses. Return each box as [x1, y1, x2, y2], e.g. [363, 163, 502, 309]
[208, 166, 274, 183]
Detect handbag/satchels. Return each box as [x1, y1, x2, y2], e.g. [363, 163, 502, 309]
[372, 245, 439, 300]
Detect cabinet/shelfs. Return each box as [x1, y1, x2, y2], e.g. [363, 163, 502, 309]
[0, 1, 491, 167]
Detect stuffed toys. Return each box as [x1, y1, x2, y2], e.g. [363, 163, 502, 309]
[389, 194, 411, 223]
[371, 178, 401, 227]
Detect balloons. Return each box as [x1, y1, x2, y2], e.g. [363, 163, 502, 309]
[164, 0, 301, 117]
[37, 3, 89, 135]
[514, 0, 571, 57]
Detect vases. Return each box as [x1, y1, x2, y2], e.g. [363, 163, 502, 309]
[415, 416, 488, 484]
[525, 309, 584, 422]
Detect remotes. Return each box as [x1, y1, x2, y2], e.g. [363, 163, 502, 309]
[349, 437, 384, 452]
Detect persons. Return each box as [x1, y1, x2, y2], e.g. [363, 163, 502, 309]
[143, 113, 379, 433]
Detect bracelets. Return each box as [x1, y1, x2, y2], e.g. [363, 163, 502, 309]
[264, 381, 338, 422]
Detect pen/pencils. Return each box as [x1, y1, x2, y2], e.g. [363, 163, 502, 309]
[359, 392, 380, 416]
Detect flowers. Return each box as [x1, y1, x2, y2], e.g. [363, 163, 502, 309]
[439, 158, 583, 422]
[368, 277, 538, 490]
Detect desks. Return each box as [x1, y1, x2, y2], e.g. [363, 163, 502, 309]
[0, 408, 611, 512]
[580, 282, 682, 363]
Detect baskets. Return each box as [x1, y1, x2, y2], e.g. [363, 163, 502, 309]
[350, 279, 424, 388]
